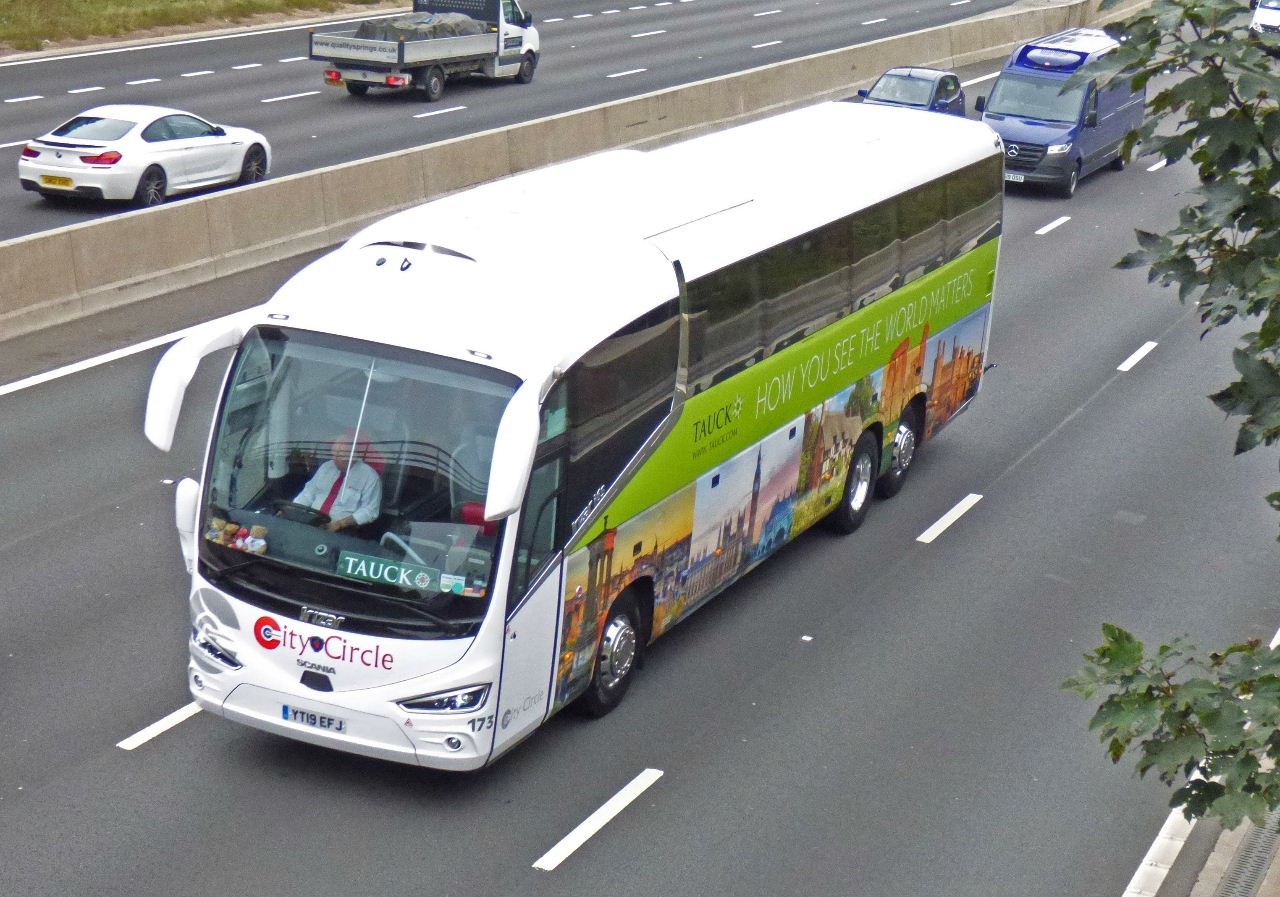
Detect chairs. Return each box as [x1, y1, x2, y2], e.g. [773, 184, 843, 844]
[327, 422, 389, 480]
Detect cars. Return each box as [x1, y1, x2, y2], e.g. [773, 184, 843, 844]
[1249, 1, 1280, 57]
[858, 66, 967, 116]
[18, 104, 272, 208]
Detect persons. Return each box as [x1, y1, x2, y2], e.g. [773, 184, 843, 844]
[278, 433, 382, 536]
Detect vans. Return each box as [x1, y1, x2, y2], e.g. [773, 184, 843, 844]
[973, 25, 1146, 200]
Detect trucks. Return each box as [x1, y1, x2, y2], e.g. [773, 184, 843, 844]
[307, 0, 541, 101]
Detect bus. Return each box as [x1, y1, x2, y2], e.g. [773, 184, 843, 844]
[144, 95, 1006, 772]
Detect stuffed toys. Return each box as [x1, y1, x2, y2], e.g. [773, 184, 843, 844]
[204, 517, 268, 556]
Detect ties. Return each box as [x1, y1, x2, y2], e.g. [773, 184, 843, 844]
[320, 472, 346, 515]
